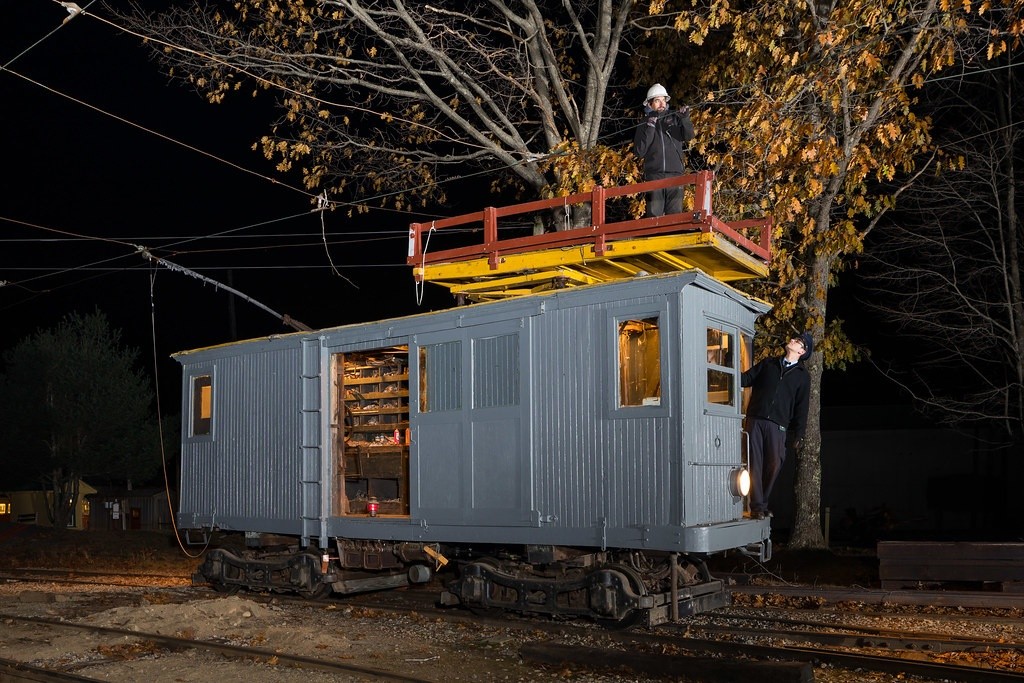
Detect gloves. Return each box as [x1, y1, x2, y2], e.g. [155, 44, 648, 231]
[646, 111, 659, 128]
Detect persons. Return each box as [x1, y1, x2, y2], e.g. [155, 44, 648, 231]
[633, 84, 694, 217]
[725, 334, 814, 519]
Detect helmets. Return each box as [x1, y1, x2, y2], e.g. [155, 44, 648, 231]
[642, 83, 671, 106]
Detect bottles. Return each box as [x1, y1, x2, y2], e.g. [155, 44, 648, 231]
[405, 428, 411, 445]
[394, 429, 399, 445]
[367, 497, 379, 517]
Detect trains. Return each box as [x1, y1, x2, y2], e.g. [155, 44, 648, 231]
[170, 175, 779, 633]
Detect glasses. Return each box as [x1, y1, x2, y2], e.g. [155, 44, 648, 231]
[793, 338, 804, 348]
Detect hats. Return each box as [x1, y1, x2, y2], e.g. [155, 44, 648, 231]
[793, 334, 813, 360]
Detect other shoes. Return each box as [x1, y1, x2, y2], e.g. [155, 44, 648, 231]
[763, 510, 773, 517]
[750, 510, 765, 520]
